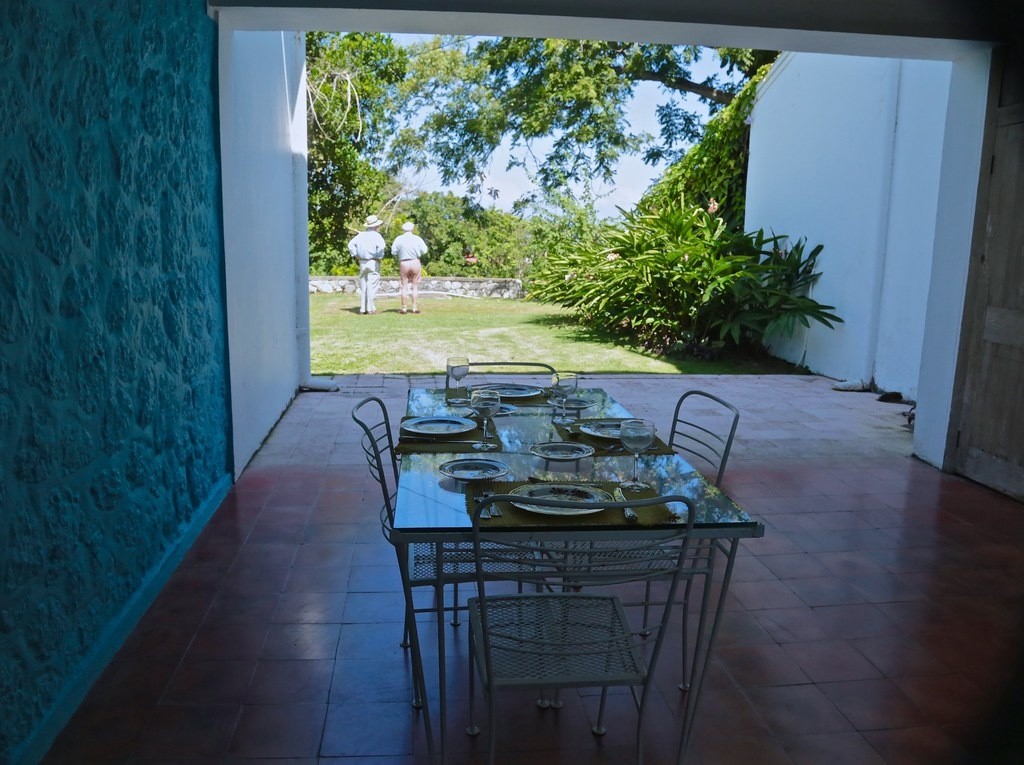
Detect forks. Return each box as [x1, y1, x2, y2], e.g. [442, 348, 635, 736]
[538, 388, 568, 398]
[472, 486, 492, 519]
[587, 440, 659, 453]
[481, 476, 503, 516]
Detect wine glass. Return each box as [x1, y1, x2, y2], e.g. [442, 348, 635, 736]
[618, 419, 656, 493]
[471, 390, 501, 451]
[551, 372, 578, 425]
[446, 357, 469, 403]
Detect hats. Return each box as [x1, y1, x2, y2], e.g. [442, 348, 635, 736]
[363, 215, 383, 227]
[402, 221, 414, 231]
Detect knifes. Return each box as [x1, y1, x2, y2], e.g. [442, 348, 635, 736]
[398, 436, 484, 444]
[614, 488, 638, 521]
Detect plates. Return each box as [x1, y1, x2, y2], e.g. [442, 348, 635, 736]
[401, 415, 478, 435]
[478, 383, 541, 399]
[580, 420, 658, 439]
[437, 458, 511, 484]
[547, 397, 592, 410]
[508, 481, 614, 516]
[469, 402, 519, 417]
[529, 441, 596, 462]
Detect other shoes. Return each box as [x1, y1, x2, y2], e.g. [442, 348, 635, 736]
[360, 311, 365, 314]
[412, 308, 420, 314]
[400, 307, 407, 314]
[367, 310, 377, 315]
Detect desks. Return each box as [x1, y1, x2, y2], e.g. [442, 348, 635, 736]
[393, 387, 764, 765]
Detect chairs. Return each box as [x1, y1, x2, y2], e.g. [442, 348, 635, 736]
[352, 362, 741, 765]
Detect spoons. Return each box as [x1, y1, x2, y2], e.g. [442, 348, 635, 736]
[475, 417, 494, 438]
[528, 476, 604, 488]
[562, 425, 582, 438]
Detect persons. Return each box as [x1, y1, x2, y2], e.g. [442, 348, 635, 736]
[391, 222, 428, 314]
[348, 215, 387, 316]
